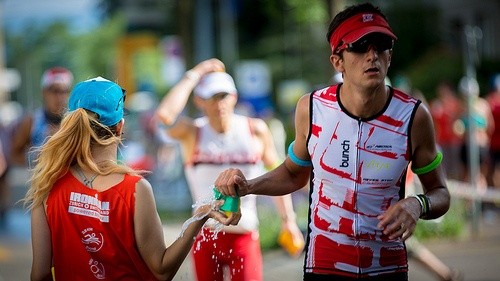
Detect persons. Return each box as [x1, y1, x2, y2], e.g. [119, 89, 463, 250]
[0, 61, 499, 223]
[214, 2, 451, 281]
[155, 56, 304, 281]
[16, 73, 241, 281]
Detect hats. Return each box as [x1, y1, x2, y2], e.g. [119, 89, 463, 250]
[68, 76, 127, 127]
[192, 71, 236, 98]
[330, 11, 399, 55]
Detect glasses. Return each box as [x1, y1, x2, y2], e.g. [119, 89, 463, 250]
[335, 32, 394, 54]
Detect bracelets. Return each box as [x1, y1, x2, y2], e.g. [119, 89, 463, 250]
[412, 194, 432, 219]
